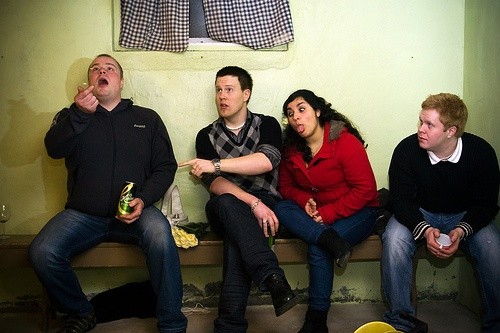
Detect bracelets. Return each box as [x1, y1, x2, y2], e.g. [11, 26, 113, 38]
[251, 199, 262, 212]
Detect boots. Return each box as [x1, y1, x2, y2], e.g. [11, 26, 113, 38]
[318, 227, 353, 276]
[297, 307, 329, 333]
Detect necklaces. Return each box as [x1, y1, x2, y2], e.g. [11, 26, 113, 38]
[312, 147, 319, 158]
[226, 122, 246, 130]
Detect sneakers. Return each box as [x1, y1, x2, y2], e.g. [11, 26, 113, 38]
[264, 273, 305, 318]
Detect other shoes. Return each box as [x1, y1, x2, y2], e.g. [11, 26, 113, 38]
[60, 310, 97, 333]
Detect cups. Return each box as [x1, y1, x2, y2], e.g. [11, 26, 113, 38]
[435, 233, 452, 253]
[353, 320, 398, 333]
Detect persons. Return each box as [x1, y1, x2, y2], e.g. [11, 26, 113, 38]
[381, 93, 500, 333]
[178, 67, 300, 333]
[26, 54, 188, 333]
[276, 89, 378, 333]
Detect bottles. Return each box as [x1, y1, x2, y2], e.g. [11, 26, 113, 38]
[263, 223, 278, 259]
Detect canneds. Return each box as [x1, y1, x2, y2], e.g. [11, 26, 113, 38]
[116, 181, 137, 216]
[267, 225, 275, 252]
[436, 233, 452, 250]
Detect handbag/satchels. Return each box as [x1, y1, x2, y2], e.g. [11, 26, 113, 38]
[160, 183, 189, 225]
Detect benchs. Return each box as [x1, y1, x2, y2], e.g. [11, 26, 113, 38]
[0, 232, 467, 317]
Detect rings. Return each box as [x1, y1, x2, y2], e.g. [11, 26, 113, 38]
[263, 222, 267, 224]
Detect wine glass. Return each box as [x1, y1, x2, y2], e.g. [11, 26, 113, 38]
[0, 200, 10, 238]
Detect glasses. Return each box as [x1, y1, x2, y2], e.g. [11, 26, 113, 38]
[88, 66, 121, 78]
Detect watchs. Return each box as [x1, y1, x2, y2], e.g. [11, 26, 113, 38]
[212, 159, 221, 175]
